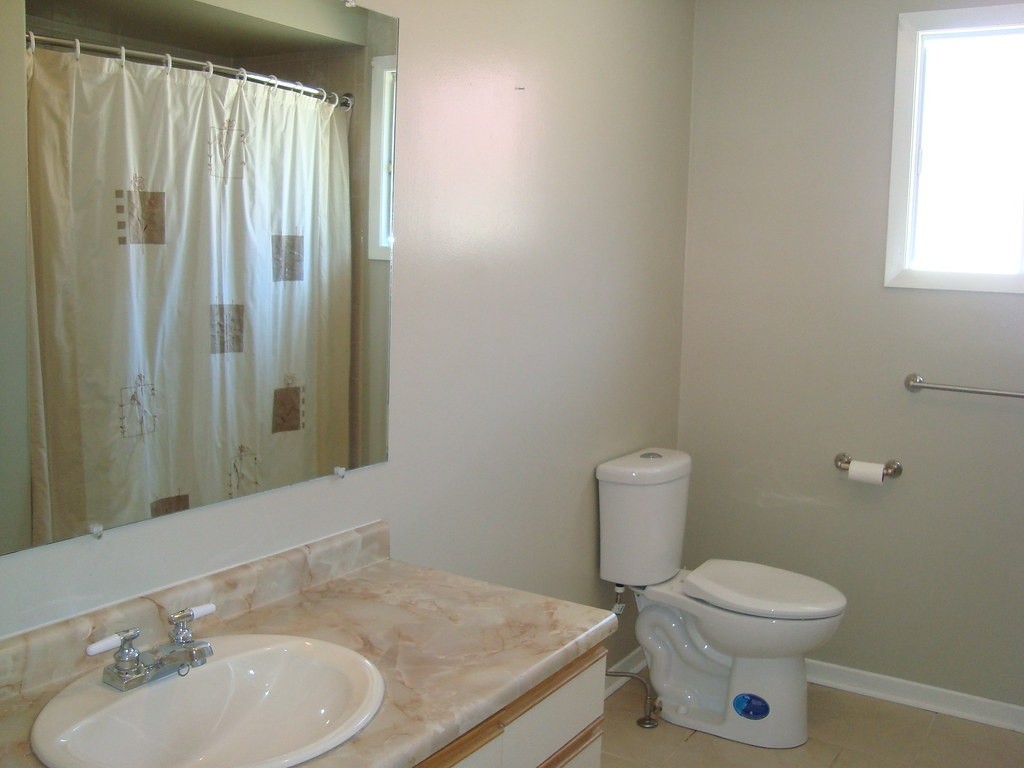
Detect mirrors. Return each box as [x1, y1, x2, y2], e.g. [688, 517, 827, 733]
[0, 0, 400, 556]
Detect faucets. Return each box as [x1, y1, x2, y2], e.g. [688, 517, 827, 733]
[85, 602, 216, 693]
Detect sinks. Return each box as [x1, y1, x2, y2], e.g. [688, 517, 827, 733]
[29, 633, 386, 768]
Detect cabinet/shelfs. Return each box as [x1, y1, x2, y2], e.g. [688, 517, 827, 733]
[411, 646, 608, 768]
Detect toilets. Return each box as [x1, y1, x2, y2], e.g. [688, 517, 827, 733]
[595, 444, 849, 749]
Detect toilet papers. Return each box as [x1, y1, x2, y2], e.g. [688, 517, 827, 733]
[848, 459, 886, 486]
